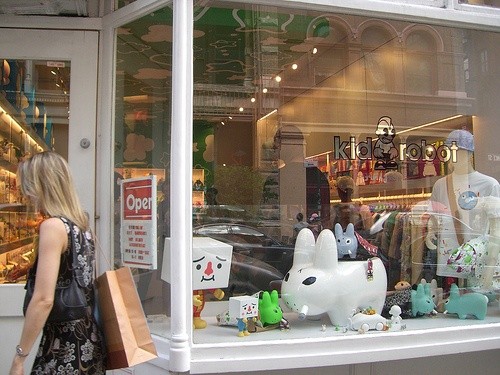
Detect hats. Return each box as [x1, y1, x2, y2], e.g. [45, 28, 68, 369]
[334, 176, 355, 194]
[443, 129, 476, 152]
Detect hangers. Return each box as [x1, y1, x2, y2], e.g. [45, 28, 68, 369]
[373, 196, 412, 213]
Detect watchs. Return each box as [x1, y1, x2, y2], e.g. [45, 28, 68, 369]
[16, 345, 29, 357]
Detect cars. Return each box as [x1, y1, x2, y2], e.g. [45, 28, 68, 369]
[191, 198, 500, 299]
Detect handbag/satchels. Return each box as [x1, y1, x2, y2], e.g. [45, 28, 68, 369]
[23, 220, 88, 322]
[92, 265, 158, 370]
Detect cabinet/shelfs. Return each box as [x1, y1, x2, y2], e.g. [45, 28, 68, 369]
[113, 166, 205, 213]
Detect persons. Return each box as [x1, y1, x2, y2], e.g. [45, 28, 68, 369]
[425, 130, 500, 280]
[8, 151, 108, 375]
[140, 126, 367, 312]
